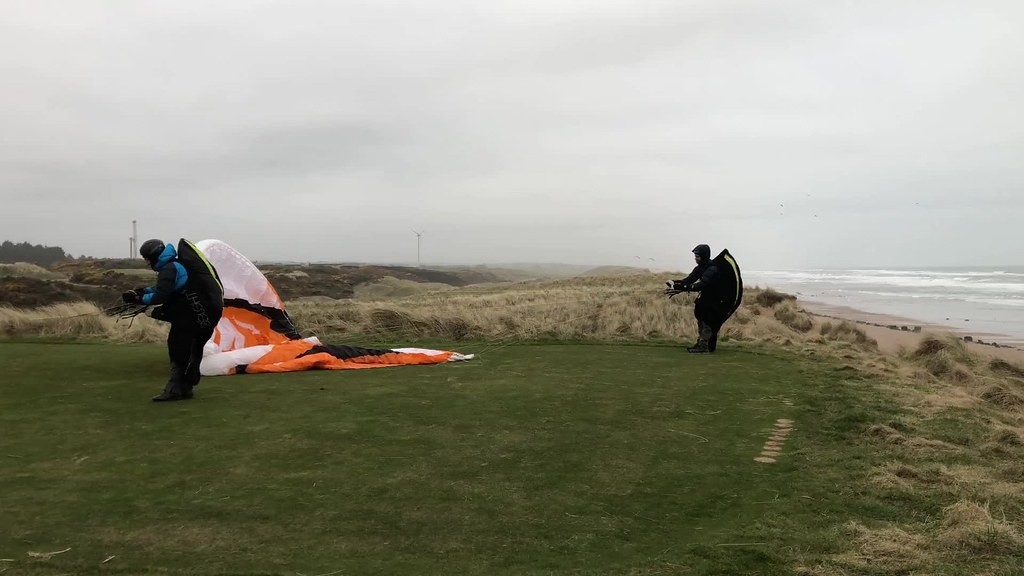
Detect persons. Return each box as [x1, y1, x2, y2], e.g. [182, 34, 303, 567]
[123, 238, 214, 402]
[666, 244, 724, 352]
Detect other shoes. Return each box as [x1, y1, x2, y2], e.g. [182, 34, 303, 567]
[152, 381, 193, 401]
[688, 337, 709, 353]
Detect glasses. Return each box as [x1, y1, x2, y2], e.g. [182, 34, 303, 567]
[694, 255, 700, 258]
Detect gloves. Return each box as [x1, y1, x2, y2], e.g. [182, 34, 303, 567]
[122, 286, 145, 305]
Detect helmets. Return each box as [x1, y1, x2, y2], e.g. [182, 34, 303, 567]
[141, 239, 166, 260]
